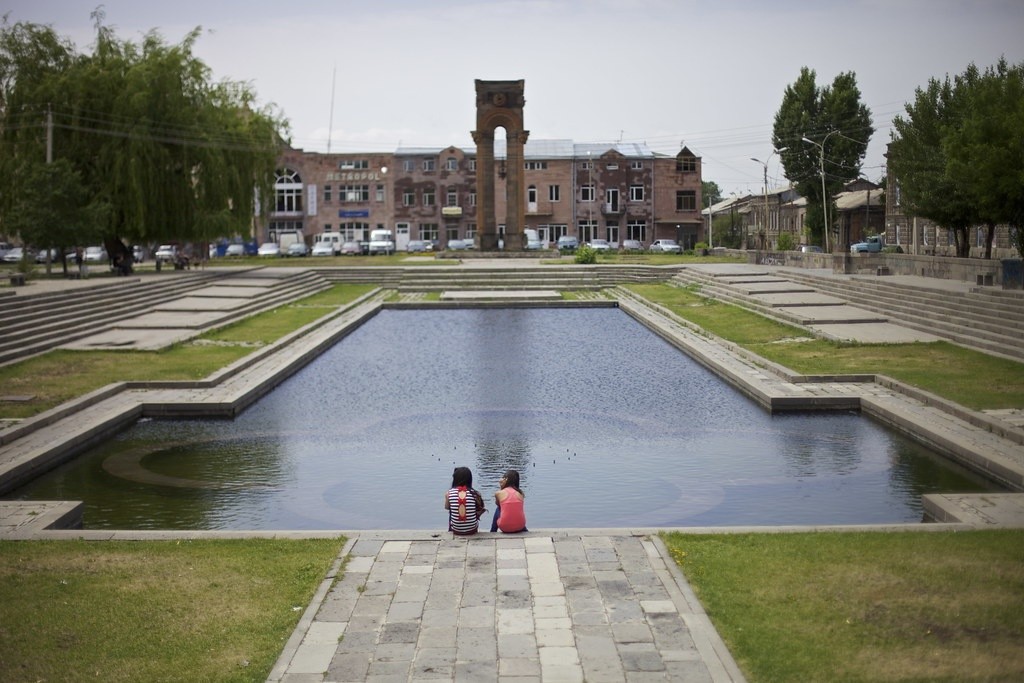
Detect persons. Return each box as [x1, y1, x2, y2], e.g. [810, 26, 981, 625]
[489, 470, 529, 534]
[445, 466, 486, 535]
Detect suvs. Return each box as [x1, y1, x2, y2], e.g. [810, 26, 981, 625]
[850, 235, 883, 253]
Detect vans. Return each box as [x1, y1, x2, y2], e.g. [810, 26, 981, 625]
[367, 229, 395, 255]
[322, 232, 344, 253]
[523, 229, 544, 250]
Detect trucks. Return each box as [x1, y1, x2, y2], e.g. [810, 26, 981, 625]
[279, 229, 305, 257]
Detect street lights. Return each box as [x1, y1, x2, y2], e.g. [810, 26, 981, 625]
[750, 146, 789, 245]
[802, 128, 839, 253]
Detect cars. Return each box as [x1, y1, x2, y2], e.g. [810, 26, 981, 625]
[132, 245, 144, 263]
[225, 244, 248, 258]
[311, 242, 336, 256]
[558, 235, 581, 250]
[286, 242, 310, 256]
[405, 240, 427, 253]
[155, 245, 186, 262]
[586, 238, 612, 251]
[258, 242, 280, 256]
[797, 245, 823, 252]
[359, 241, 369, 254]
[37, 249, 56, 263]
[0, 241, 25, 263]
[66, 254, 78, 263]
[648, 238, 684, 254]
[622, 239, 644, 251]
[82, 245, 108, 264]
[424, 238, 475, 252]
[339, 241, 362, 257]
